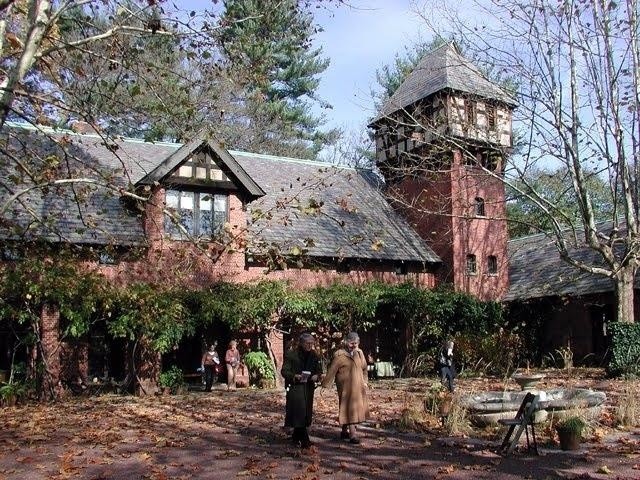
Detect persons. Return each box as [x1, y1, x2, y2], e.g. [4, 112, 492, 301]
[281, 332, 325, 450]
[319, 333, 370, 444]
[436, 340, 456, 393]
[200, 344, 220, 392]
[224, 340, 240, 392]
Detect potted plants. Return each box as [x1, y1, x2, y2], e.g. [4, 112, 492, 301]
[554, 416, 586, 451]
[159, 364, 184, 395]
[0, 378, 29, 407]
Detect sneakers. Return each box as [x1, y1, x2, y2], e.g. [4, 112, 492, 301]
[340, 430, 362, 444]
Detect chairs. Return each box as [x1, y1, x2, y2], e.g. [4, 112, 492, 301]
[65, 373, 136, 400]
[496, 392, 540, 458]
